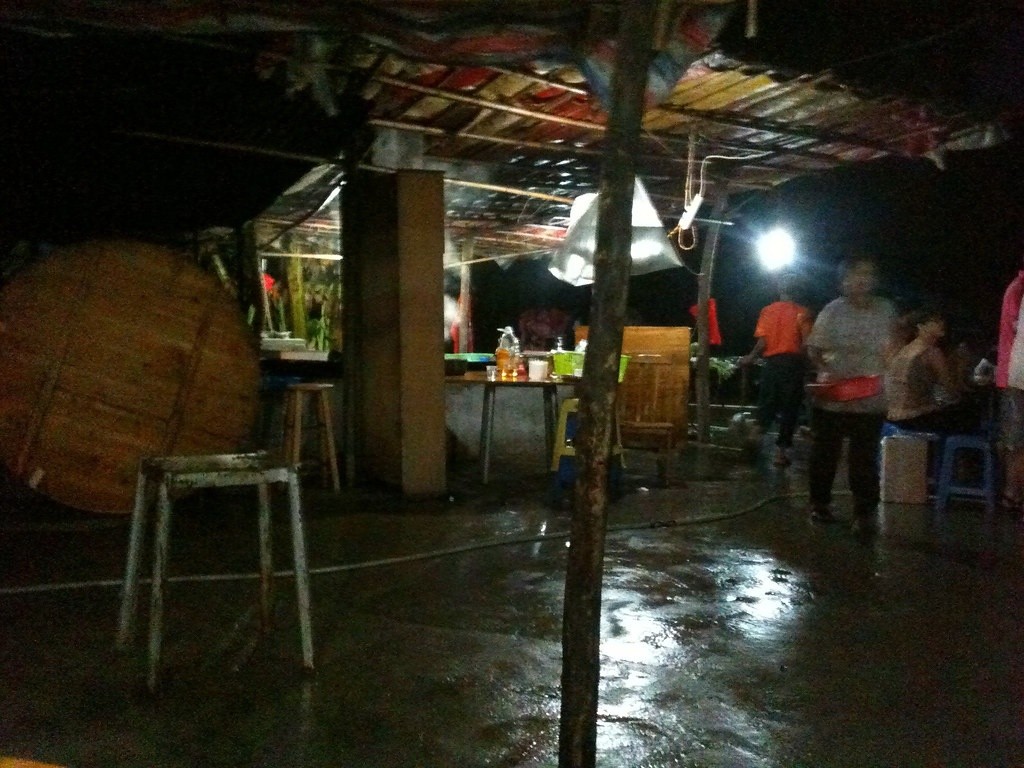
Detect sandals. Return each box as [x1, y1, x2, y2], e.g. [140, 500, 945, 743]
[998, 494, 1022, 511]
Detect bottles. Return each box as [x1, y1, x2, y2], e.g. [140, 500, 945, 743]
[495, 326, 520, 382]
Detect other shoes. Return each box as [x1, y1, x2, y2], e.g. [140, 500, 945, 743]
[747, 424, 763, 447]
[810, 503, 833, 522]
[775, 445, 795, 463]
[852, 515, 878, 536]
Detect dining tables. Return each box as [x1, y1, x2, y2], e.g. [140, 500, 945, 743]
[447, 372, 582, 485]
[0, 239, 259, 512]
[575, 325, 691, 447]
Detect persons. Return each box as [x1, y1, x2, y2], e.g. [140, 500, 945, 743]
[735, 274, 814, 466]
[804, 254, 899, 535]
[889, 268, 1024, 517]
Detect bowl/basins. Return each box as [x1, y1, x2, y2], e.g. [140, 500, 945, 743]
[550, 350, 631, 384]
[803, 374, 881, 401]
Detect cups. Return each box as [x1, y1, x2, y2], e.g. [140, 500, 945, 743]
[528, 360, 548, 379]
[486, 365, 497, 382]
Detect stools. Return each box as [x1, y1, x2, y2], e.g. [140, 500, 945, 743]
[550, 398, 626, 471]
[935, 434, 996, 518]
[877, 419, 946, 503]
[116, 448, 317, 693]
[278, 382, 340, 494]
